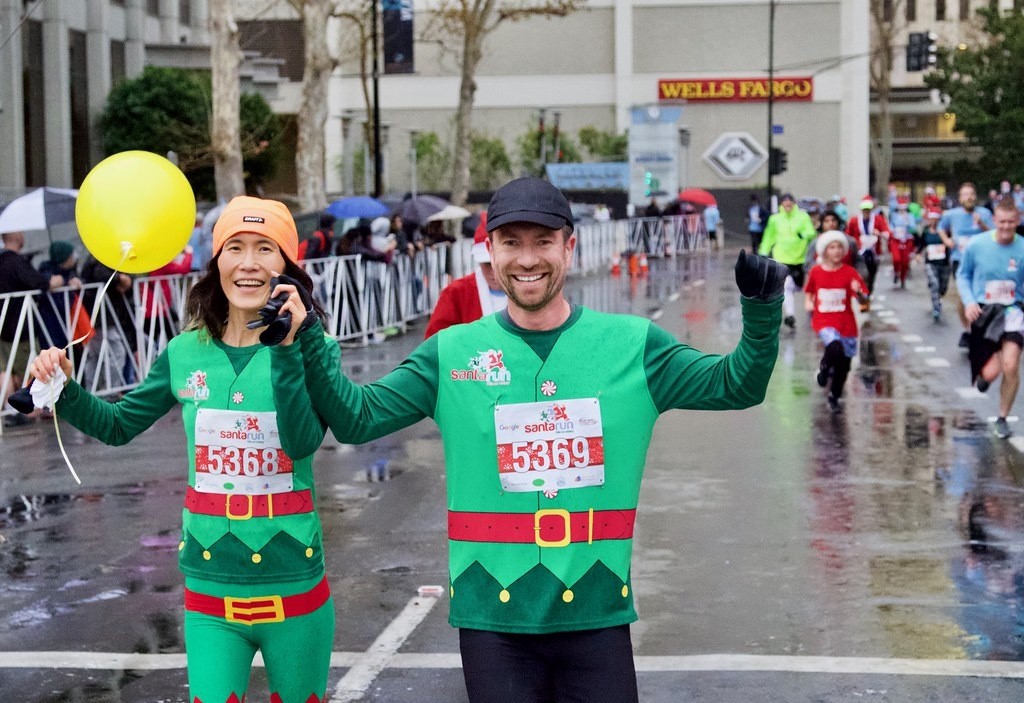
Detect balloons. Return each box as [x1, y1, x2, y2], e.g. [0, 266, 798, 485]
[75, 150, 199, 275]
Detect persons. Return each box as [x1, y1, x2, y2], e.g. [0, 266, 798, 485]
[954, 201, 1024, 439]
[799, 183, 952, 323]
[0, 231, 54, 425]
[423, 212, 510, 340]
[643, 197, 720, 256]
[743, 193, 770, 257]
[935, 181, 996, 330]
[757, 194, 818, 329]
[594, 203, 610, 222]
[187, 212, 202, 320]
[297, 214, 457, 350]
[803, 229, 873, 412]
[21, 196, 340, 703]
[983, 180, 1024, 237]
[38, 238, 193, 403]
[267, 179, 790, 703]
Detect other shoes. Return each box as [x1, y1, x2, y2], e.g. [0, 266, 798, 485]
[976, 373, 990, 394]
[816, 364, 828, 388]
[993, 419, 1011, 439]
[825, 391, 839, 413]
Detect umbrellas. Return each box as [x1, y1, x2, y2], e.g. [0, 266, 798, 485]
[392, 194, 449, 221]
[324, 195, 391, 217]
[428, 204, 473, 221]
[0, 182, 85, 254]
[678, 190, 718, 206]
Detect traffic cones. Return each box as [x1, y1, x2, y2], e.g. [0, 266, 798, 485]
[638, 251, 650, 273]
[629, 255, 641, 274]
[612, 252, 621, 279]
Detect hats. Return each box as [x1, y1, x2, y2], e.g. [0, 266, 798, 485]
[213, 195, 299, 266]
[471, 211, 491, 264]
[814, 231, 849, 258]
[486, 176, 575, 234]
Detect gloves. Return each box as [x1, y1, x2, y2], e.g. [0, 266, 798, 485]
[273, 272, 317, 333]
[246, 277, 292, 347]
[7, 373, 60, 414]
[735, 248, 789, 299]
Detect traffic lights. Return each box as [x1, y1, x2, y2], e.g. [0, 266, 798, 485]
[773, 147, 788, 175]
[922, 30, 938, 66]
